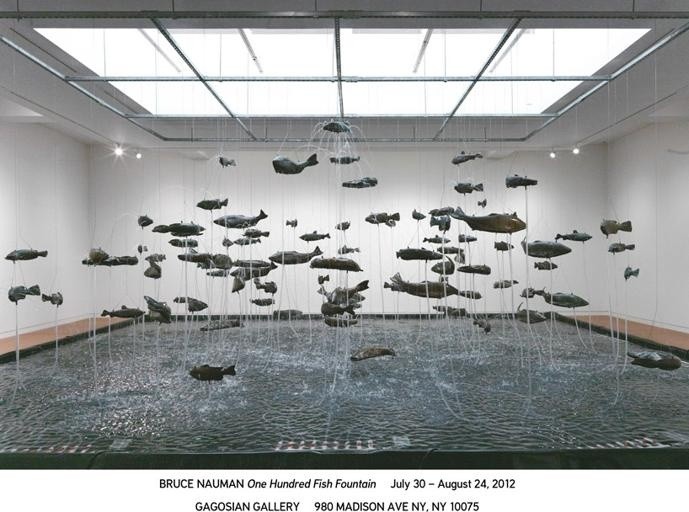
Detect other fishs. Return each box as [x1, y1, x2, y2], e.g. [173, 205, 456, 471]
[81, 198, 592, 335]
